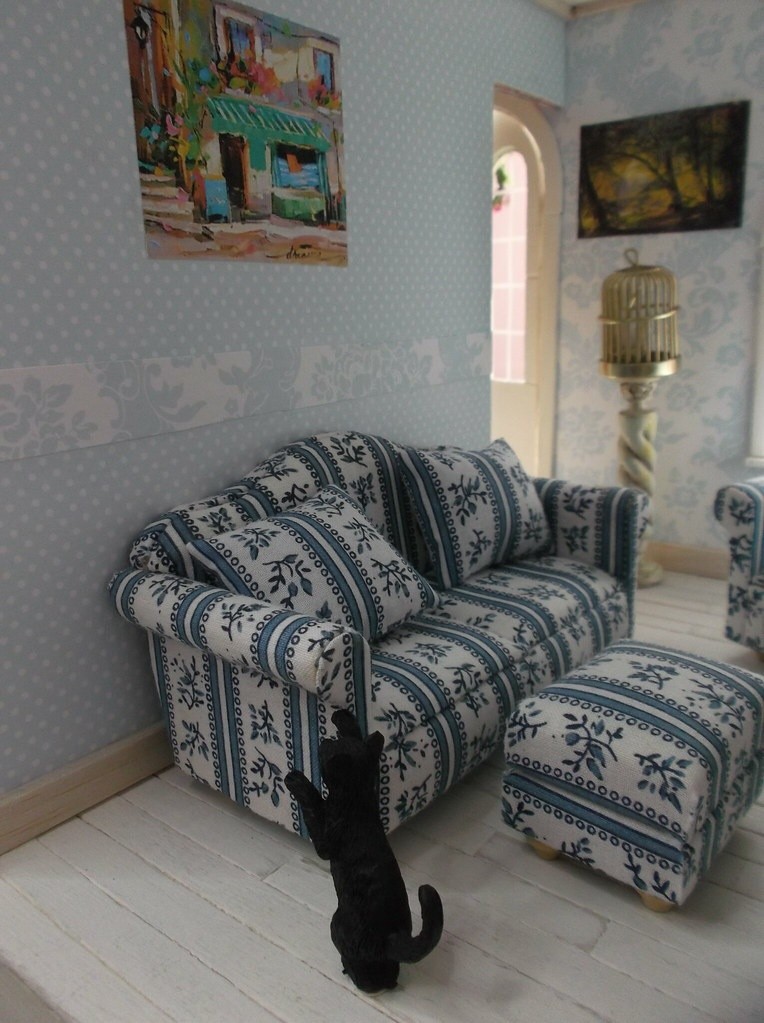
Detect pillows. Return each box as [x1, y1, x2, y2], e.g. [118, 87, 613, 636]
[387, 440, 557, 593]
[185, 484, 445, 657]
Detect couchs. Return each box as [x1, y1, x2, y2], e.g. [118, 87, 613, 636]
[116, 426, 647, 839]
[713, 476, 764, 658]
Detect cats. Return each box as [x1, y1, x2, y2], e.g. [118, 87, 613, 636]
[284, 708, 444, 996]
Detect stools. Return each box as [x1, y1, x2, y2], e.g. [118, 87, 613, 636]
[501, 635, 764, 911]
